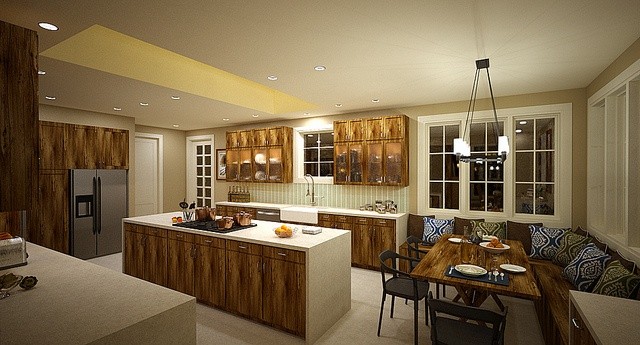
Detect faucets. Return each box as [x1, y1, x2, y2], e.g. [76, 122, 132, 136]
[305, 173, 315, 207]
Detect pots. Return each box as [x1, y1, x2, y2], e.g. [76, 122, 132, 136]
[233, 211, 252, 226]
[195, 206, 216, 221]
[215, 217, 233, 230]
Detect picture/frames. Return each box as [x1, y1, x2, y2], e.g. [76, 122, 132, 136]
[215, 148, 226, 181]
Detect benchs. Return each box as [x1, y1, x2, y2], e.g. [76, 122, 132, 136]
[531, 261, 589, 345]
[399, 240, 552, 283]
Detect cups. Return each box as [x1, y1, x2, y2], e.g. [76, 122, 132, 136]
[463, 225, 470, 237]
[183, 210, 194, 221]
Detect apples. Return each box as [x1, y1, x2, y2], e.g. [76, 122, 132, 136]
[487, 239, 504, 248]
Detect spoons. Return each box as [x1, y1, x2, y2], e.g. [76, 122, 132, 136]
[500, 273, 504, 281]
[449, 266, 455, 274]
[493, 270, 499, 282]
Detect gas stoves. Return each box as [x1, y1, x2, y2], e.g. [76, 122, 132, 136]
[172, 219, 257, 234]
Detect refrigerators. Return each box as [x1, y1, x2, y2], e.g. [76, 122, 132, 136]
[68, 169, 127, 259]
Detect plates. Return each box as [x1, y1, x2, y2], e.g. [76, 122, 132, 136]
[455, 265, 488, 276]
[500, 264, 527, 273]
[448, 238, 464, 244]
[479, 242, 510, 253]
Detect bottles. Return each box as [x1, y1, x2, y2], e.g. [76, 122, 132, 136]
[228, 186, 232, 202]
[461, 240, 474, 263]
[246, 186, 250, 203]
[239, 186, 242, 202]
[231, 186, 236, 202]
[236, 186, 239, 203]
[242, 186, 246, 203]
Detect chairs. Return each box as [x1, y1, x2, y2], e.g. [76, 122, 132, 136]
[429, 291, 509, 345]
[406, 234, 435, 270]
[377, 250, 429, 344]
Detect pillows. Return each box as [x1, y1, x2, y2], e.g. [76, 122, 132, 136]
[505, 220, 543, 255]
[522, 203, 540, 214]
[552, 230, 593, 266]
[560, 241, 612, 290]
[593, 259, 640, 299]
[422, 216, 454, 245]
[453, 217, 485, 235]
[471, 221, 506, 241]
[529, 225, 572, 260]
[406, 213, 436, 241]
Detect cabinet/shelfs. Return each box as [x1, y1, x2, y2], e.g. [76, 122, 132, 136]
[365, 116, 409, 187]
[238, 201, 257, 221]
[353, 209, 408, 273]
[68, 126, 128, 170]
[0, 20, 39, 212]
[226, 210, 352, 344]
[27, 120, 69, 257]
[566, 290, 640, 344]
[251, 127, 293, 183]
[318, 203, 352, 235]
[257, 202, 280, 222]
[333, 119, 365, 185]
[225, 130, 252, 182]
[215, 201, 238, 217]
[169, 210, 227, 315]
[122, 212, 169, 289]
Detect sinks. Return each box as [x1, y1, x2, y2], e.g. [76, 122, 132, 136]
[280, 205, 318, 224]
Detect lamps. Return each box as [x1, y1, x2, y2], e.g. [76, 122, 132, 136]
[452, 58, 510, 164]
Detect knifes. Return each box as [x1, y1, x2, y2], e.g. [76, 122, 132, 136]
[488, 271, 492, 281]
